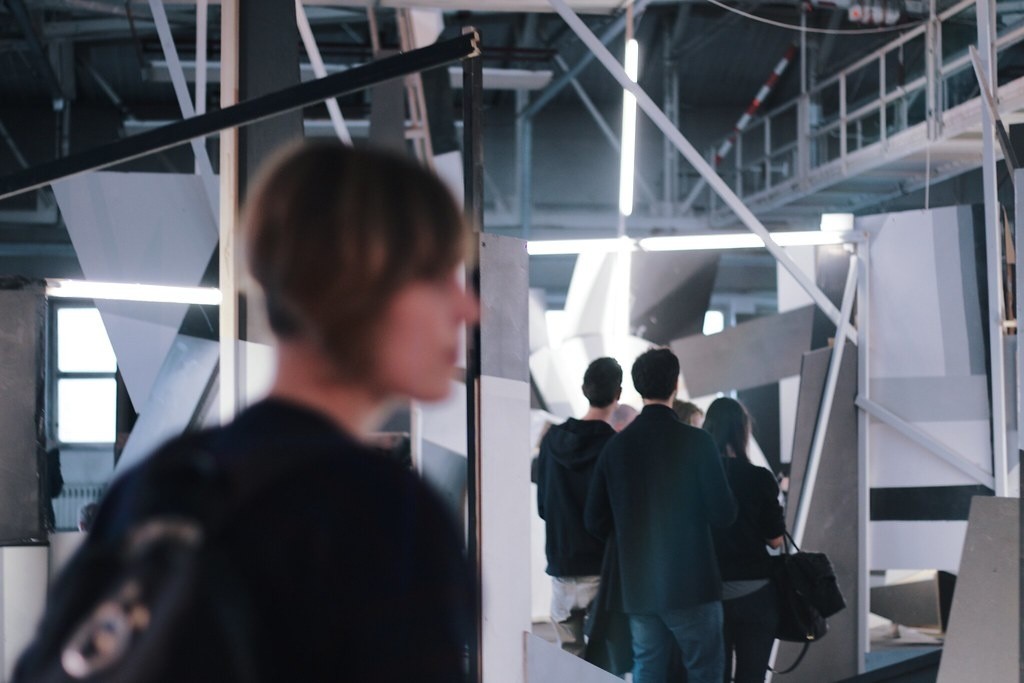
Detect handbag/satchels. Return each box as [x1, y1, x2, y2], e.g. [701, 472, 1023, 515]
[763, 527, 844, 674]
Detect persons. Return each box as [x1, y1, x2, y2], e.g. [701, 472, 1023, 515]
[0, 136, 482, 683]
[531, 347, 784, 683]
